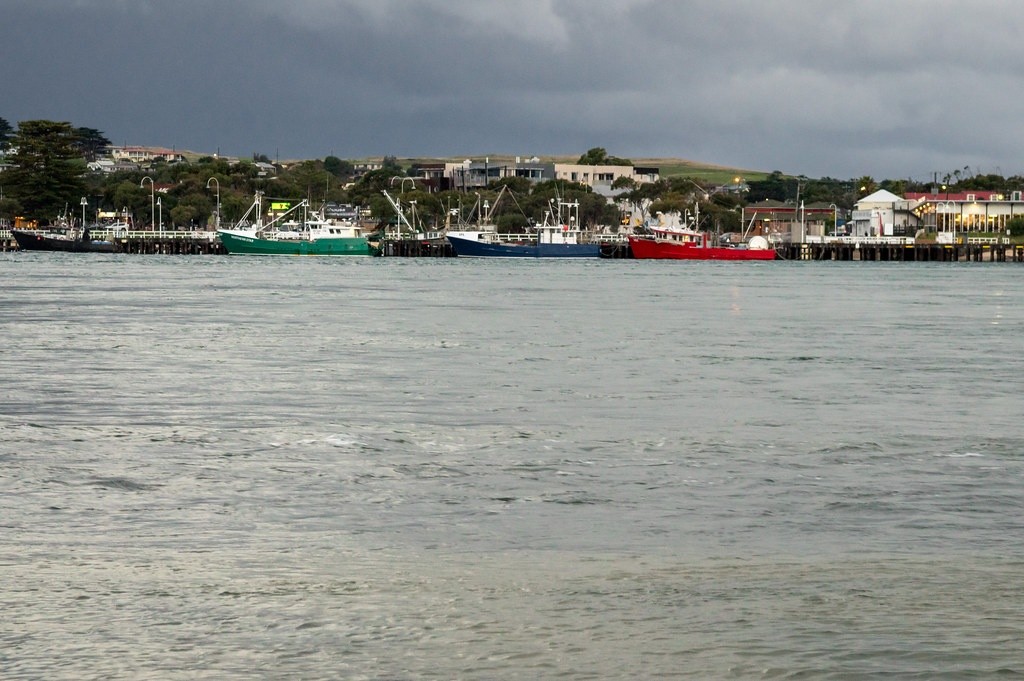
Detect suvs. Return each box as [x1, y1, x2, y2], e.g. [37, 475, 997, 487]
[105, 223, 129, 232]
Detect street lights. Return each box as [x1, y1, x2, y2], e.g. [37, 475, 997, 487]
[829, 204, 836, 239]
[123, 206, 127, 232]
[80, 197, 88, 230]
[206, 177, 219, 230]
[396, 198, 401, 240]
[799, 200, 804, 243]
[549, 194, 554, 221]
[391, 177, 416, 194]
[567, 201, 571, 232]
[156, 197, 162, 239]
[694, 202, 700, 234]
[946, 201, 955, 245]
[140, 177, 154, 232]
[684, 208, 691, 231]
[935, 203, 945, 243]
[473, 191, 481, 227]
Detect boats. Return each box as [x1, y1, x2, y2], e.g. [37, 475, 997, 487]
[444, 195, 600, 261]
[217, 189, 369, 255]
[628, 225, 775, 260]
[10, 227, 116, 252]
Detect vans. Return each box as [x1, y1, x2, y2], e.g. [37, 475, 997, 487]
[278, 223, 302, 235]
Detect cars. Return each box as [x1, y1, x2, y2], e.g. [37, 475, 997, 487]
[365, 233, 386, 241]
[719, 231, 735, 242]
[828, 228, 848, 236]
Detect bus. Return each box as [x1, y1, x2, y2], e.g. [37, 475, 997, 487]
[592, 235, 624, 242]
